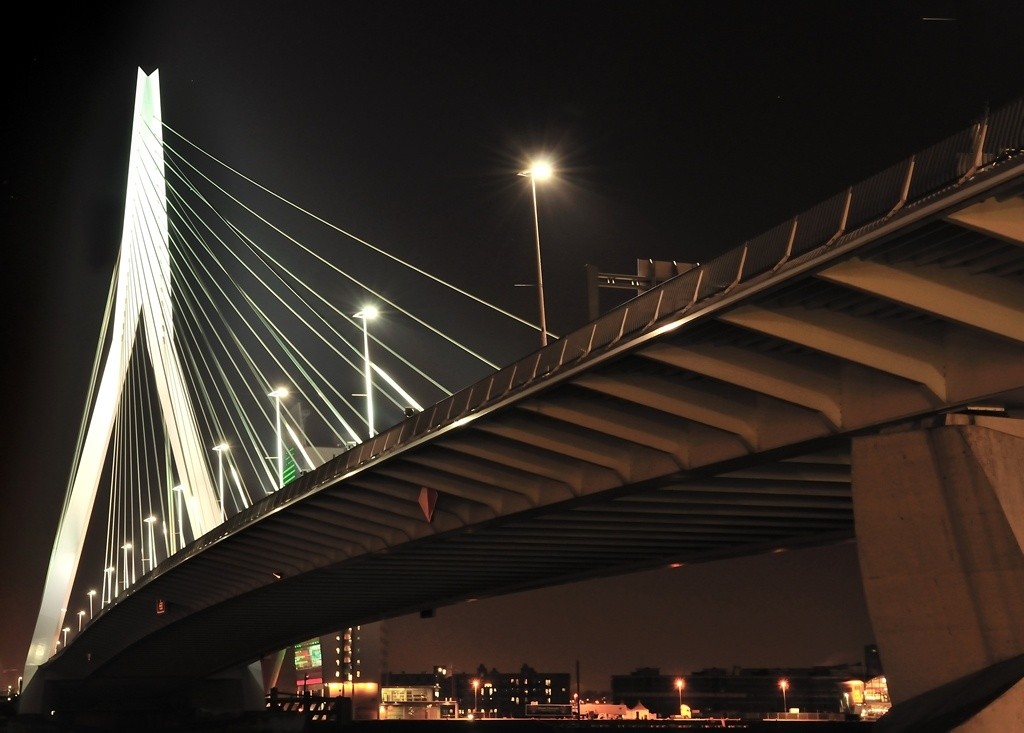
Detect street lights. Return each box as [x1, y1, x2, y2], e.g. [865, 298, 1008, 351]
[143, 516, 157, 571]
[173, 484, 188, 550]
[779, 680, 789, 712]
[77, 610, 88, 632]
[212, 443, 229, 526]
[61, 627, 72, 647]
[121, 542, 133, 588]
[269, 388, 289, 489]
[86, 589, 97, 620]
[54, 641, 61, 656]
[472, 679, 480, 713]
[675, 679, 685, 715]
[351, 303, 380, 439]
[516, 162, 553, 348]
[104, 566, 115, 604]
